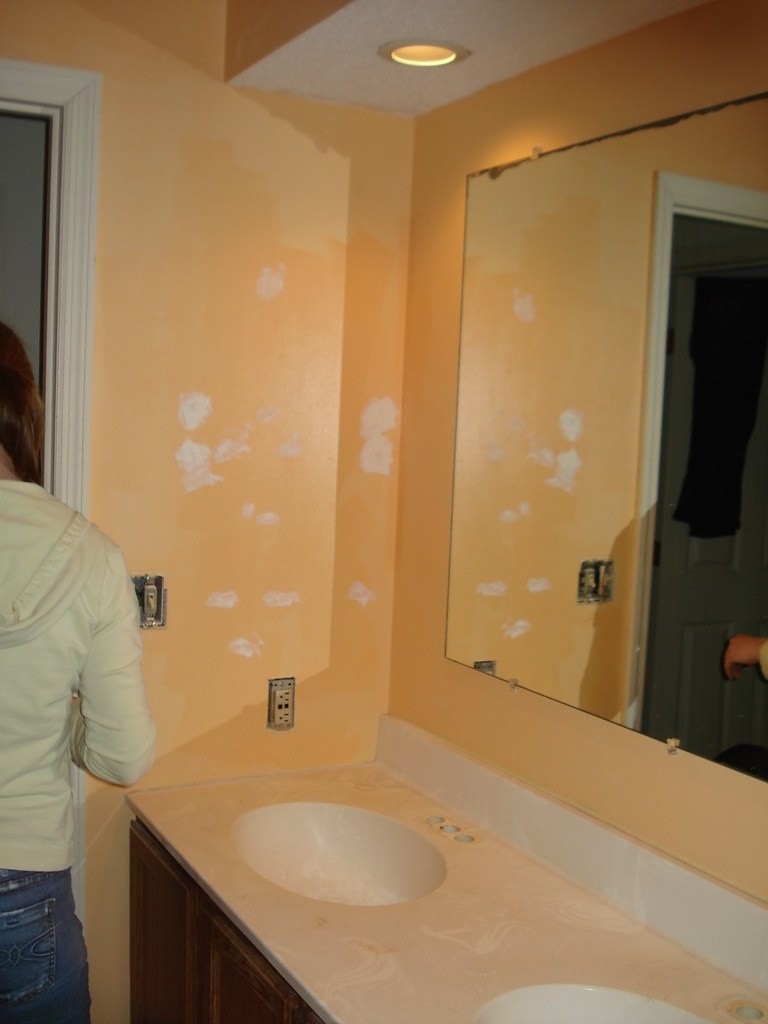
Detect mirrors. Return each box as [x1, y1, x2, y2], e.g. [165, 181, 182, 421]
[444, 91, 768, 787]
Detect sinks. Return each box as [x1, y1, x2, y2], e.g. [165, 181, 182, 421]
[472, 982, 711, 1023]
[228, 797, 450, 909]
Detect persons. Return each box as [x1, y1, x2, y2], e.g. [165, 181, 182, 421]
[724, 635, 768, 680]
[0, 320, 155, 1024]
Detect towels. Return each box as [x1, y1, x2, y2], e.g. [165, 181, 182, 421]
[671, 273, 768, 541]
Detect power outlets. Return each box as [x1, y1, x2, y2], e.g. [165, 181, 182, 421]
[267, 677, 296, 729]
[473, 660, 497, 677]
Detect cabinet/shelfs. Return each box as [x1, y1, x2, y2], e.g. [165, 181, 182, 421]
[129, 817, 324, 1024]
